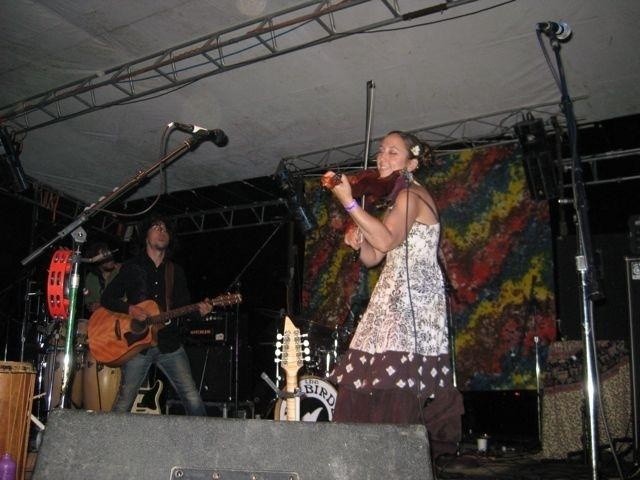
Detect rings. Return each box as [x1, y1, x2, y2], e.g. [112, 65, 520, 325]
[202, 311, 204, 314]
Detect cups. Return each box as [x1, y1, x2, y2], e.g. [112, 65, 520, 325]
[477, 437, 487, 452]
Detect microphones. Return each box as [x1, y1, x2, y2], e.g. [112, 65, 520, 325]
[536, 21, 572, 40]
[172, 121, 228, 148]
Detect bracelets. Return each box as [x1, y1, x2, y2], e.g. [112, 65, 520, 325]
[344, 199, 358, 212]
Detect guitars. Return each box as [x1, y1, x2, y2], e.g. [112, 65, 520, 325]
[274, 316, 311, 421]
[88, 291, 242, 367]
[129, 360, 163, 415]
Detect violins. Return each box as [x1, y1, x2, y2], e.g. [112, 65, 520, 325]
[321, 164, 408, 209]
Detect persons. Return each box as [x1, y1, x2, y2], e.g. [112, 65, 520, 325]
[100, 214, 214, 417]
[321, 130, 465, 460]
[79, 244, 128, 314]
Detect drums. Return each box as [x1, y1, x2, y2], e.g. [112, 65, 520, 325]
[271, 373, 339, 421]
[0, 361, 37, 479]
[278, 317, 336, 371]
[315, 347, 350, 383]
[43, 318, 122, 412]
